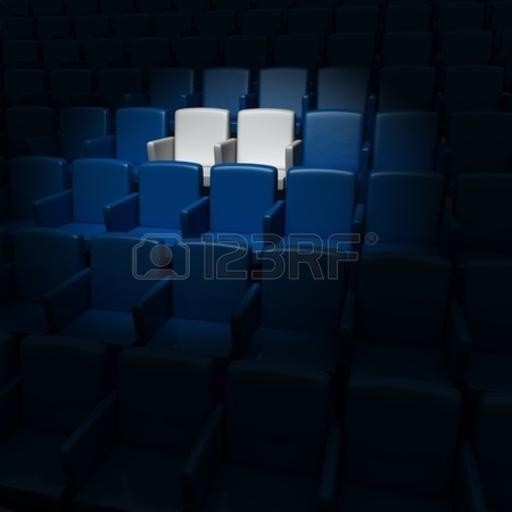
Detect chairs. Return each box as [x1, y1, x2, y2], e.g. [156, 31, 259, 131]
[1, 2, 512, 512]
